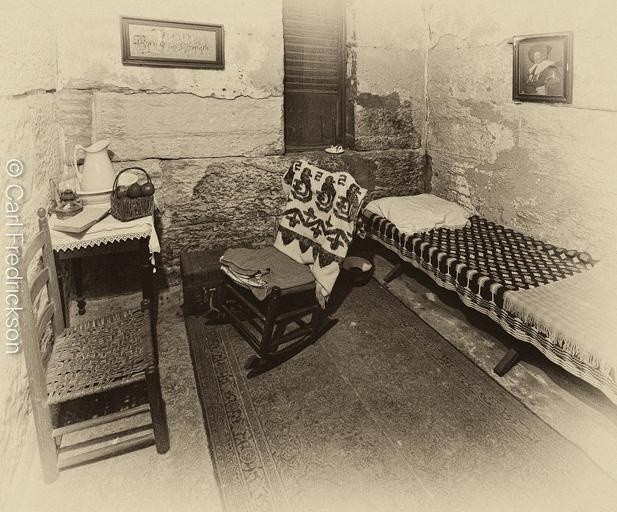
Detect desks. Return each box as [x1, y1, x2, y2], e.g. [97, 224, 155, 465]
[45, 205, 161, 359]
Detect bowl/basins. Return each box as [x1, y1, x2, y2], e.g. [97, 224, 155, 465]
[57, 170, 138, 205]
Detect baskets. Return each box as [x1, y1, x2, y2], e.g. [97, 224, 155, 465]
[109, 167, 155, 221]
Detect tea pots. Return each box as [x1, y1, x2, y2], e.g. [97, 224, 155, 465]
[73, 139, 118, 190]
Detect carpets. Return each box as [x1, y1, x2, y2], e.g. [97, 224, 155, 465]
[182, 279, 617, 511]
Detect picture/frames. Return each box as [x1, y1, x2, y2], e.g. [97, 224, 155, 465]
[510, 31, 575, 104]
[118, 14, 225, 71]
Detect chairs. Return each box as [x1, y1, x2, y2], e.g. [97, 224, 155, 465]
[200, 152, 374, 373]
[14, 207, 172, 484]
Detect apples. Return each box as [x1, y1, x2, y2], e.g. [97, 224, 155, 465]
[116, 183, 155, 205]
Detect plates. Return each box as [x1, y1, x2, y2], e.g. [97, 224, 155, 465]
[324, 147, 345, 155]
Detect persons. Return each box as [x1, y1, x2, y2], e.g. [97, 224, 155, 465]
[520, 44, 563, 101]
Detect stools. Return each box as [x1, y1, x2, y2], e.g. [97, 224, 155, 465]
[178, 249, 234, 315]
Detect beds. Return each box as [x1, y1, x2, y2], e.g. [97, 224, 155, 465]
[360, 151, 617, 408]
